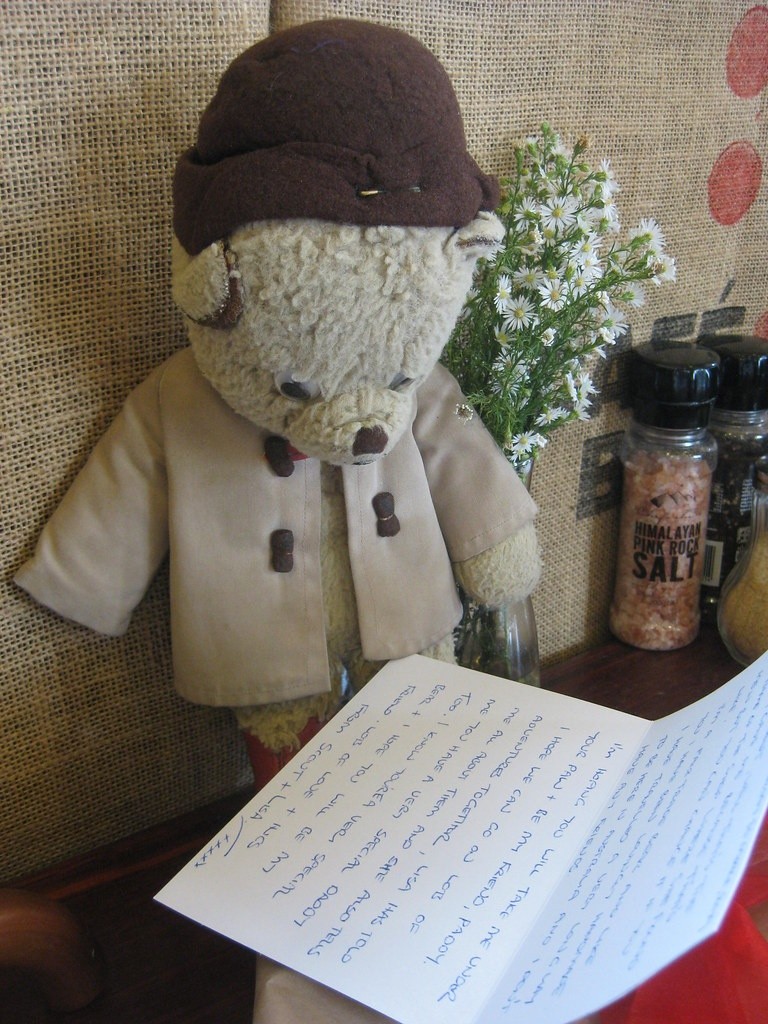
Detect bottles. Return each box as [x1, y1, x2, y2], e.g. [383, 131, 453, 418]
[607, 340, 720, 651]
[716, 457, 768, 667]
[697, 334, 768, 607]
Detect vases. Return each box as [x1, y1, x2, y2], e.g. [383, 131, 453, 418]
[456, 456, 540, 689]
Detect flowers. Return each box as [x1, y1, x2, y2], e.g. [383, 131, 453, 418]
[443, 122, 680, 674]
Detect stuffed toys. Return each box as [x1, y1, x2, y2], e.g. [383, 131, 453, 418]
[12, 20, 542, 789]
